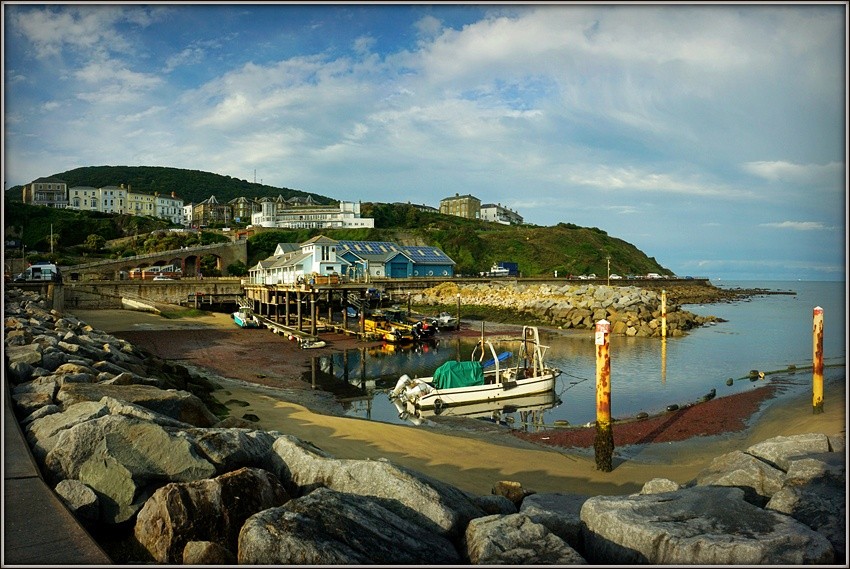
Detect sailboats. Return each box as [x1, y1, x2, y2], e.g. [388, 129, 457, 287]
[386, 325, 563, 412]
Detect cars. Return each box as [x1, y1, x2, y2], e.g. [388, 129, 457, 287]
[153, 275, 175, 281]
[577, 273, 599, 280]
[627, 273, 679, 280]
[609, 273, 622, 281]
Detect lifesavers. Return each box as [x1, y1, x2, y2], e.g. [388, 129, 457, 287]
[434, 399, 443, 409]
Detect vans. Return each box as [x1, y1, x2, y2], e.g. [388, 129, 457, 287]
[13, 264, 57, 282]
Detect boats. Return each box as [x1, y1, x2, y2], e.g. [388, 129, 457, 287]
[480, 262, 509, 278]
[232, 306, 260, 329]
[300, 340, 326, 349]
[341, 307, 458, 342]
